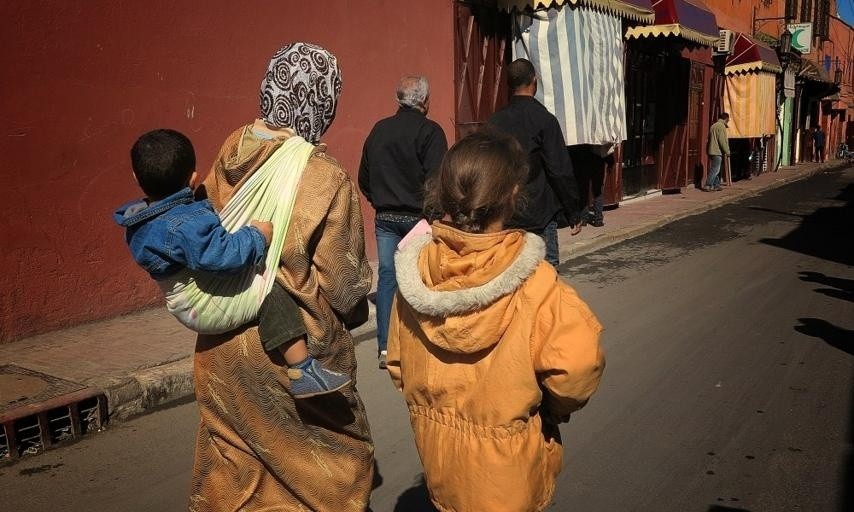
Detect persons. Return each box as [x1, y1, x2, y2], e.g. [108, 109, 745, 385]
[487, 58, 583, 271]
[702, 113, 731, 192]
[189, 41, 372, 511]
[386, 129, 606, 511]
[578, 144, 604, 227]
[359, 77, 449, 369]
[113, 129, 351, 399]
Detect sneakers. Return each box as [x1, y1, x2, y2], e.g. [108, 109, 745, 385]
[378, 355, 387, 369]
[703, 185, 723, 192]
[287, 355, 352, 399]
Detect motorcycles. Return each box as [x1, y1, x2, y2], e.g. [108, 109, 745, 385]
[836, 142, 854, 163]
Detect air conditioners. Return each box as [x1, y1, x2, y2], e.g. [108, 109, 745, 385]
[718, 29, 736, 52]
